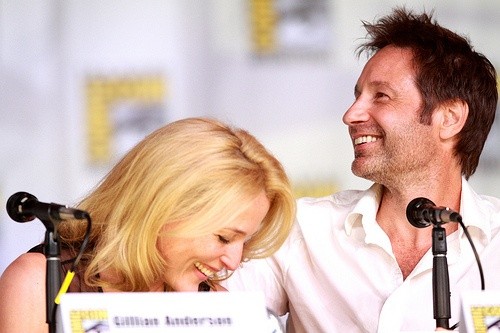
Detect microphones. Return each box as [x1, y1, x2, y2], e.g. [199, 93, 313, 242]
[406, 197, 462, 228]
[6, 192, 86, 223]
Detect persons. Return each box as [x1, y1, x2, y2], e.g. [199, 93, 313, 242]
[220, 7, 500, 333]
[0, 116, 294, 333]
